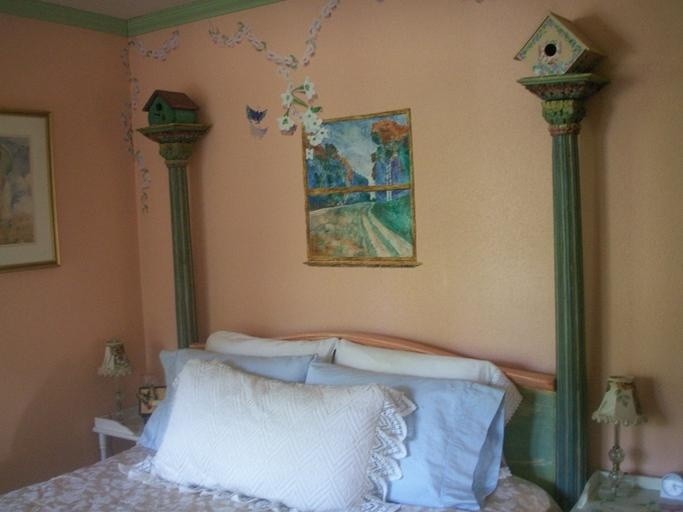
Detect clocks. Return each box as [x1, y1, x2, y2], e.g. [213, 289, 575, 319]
[659, 472, 683, 501]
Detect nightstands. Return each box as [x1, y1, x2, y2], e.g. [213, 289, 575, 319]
[568, 471, 683, 512]
[91, 404, 146, 460]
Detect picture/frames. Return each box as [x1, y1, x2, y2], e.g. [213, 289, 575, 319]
[302, 107, 421, 268]
[0, 106, 61, 271]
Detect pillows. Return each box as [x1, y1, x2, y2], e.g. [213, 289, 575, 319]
[134, 344, 321, 461]
[203, 328, 339, 362]
[303, 357, 509, 510]
[332, 337, 521, 425]
[123, 357, 421, 510]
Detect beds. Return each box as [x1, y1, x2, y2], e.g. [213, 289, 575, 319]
[0, 12, 614, 511]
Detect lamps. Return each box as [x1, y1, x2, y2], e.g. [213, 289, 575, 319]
[591, 377, 650, 499]
[96, 342, 134, 425]
